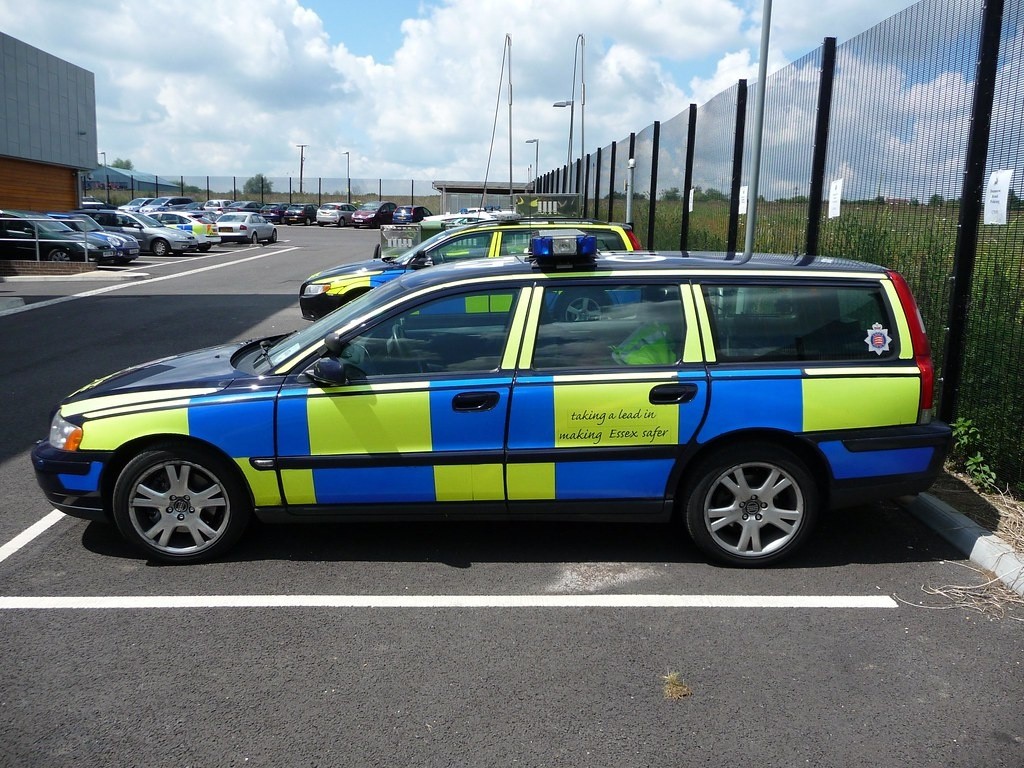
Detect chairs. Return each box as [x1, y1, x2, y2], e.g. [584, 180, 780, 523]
[792, 295, 841, 358]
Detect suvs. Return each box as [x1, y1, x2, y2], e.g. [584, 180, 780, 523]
[297, 213, 647, 332]
[202, 199, 235, 215]
[25, 229, 957, 566]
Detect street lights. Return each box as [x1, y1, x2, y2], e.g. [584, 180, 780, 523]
[553, 99, 575, 188]
[526, 138, 541, 197]
[342, 151, 350, 203]
[97, 150, 110, 203]
[296, 144, 308, 194]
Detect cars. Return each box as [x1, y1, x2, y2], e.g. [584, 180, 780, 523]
[139, 196, 193, 215]
[373, 206, 523, 266]
[350, 201, 399, 230]
[182, 202, 206, 211]
[258, 202, 296, 226]
[118, 196, 156, 211]
[81, 195, 106, 210]
[284, 204, 320, 225]
[143, 210, 222, 253]
[214, 212, 278, 244]
[316, 203, 357, 226]
[221, 200, 265, 216]
[43, 210, 140, 265]
[78, 208, 200, 257]
[391, 205, 434, 224]
[0, 208, 117, 266]
